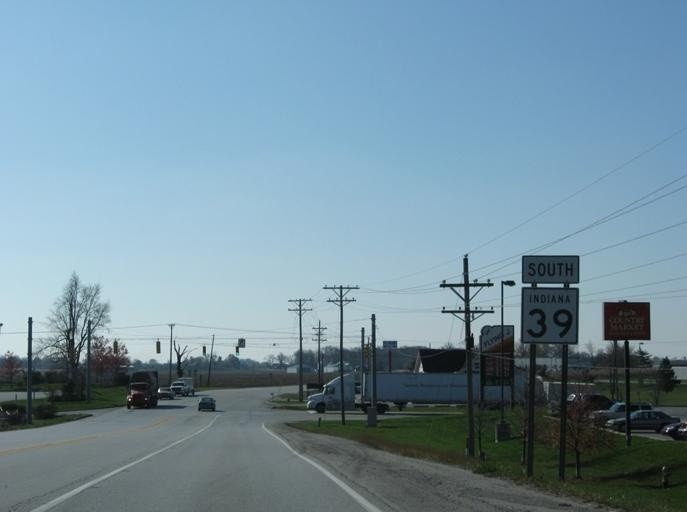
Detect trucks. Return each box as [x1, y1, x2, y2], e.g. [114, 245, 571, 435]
[126, 370, 159, 409]
[170, 377, 194, 397]
[306, 370, 546, 414]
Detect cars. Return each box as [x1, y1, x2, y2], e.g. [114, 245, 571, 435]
[197, 397, 216, 411]
[157, 387, 175, 400]
[556, 393, 687, 441]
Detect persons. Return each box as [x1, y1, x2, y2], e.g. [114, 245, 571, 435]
[661, 463, 672, 491]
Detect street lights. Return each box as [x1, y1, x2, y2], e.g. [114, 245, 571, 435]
[501, 281, 515, 426]
